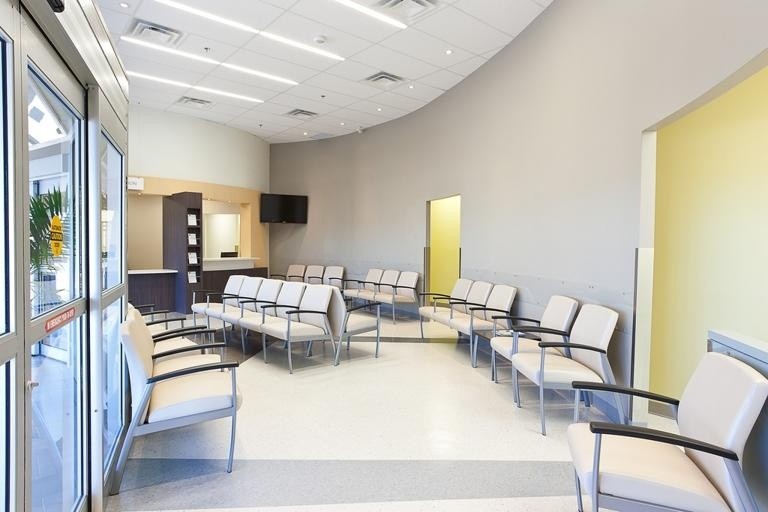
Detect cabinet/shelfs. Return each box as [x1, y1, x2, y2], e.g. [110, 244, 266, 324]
[127, 268, 178, 314]
[185, 207, 201, 283]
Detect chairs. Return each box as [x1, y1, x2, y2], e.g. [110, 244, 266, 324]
[191, 262, 380, 374]
[568, 352, 767, 512]
[344, 268, 422, 325]
[513, 305, 626, 435]
[491, 295, 587, 404]
[110, 301, 242, 496]
[417, 277, 518, 366]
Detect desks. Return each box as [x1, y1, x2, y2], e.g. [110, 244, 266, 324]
[473, 329, 541, 381]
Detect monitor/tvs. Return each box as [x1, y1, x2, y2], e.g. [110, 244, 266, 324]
[260, 193, 308, 224]
